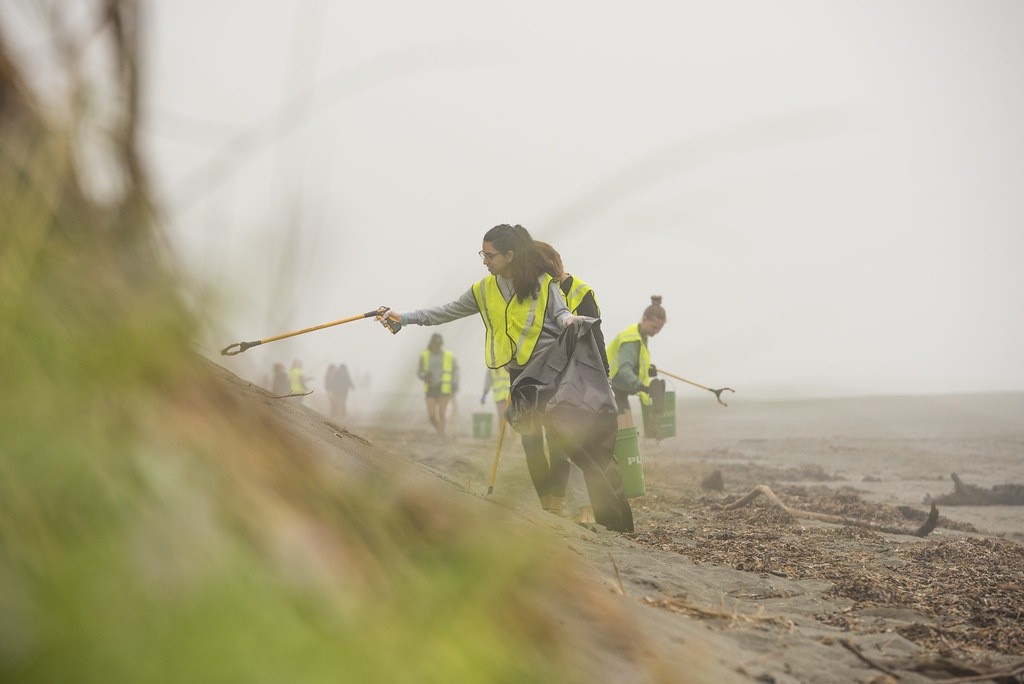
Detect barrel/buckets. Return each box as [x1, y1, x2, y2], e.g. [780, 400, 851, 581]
[613, 424, 646, 497]
[641, 373, 677, 439]
[472, 401, 493, 439]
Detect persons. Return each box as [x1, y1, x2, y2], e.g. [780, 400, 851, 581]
[532, 240, 635, 534]
[601, 295, 667, 432]
[480, 361, 513, 442]
[418, 333, 459, 435]
[288, 358, 309, 404]
[374, 224, 575, 517]
[272, 364, 291, 395]
[323, 363, 354, 421]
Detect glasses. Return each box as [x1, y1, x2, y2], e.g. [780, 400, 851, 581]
[478, 250, 503, 260]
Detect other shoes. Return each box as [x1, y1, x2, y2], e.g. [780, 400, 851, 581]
[539, 494, 567, 515]
